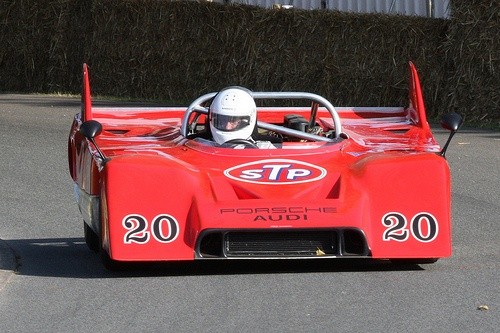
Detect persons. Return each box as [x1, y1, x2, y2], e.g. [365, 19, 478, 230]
[208, 86, 257, 147]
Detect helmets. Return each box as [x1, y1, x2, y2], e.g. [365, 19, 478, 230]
[209, 86, 257, 145]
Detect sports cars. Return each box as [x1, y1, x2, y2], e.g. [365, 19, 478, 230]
[68, 61, 465, 273]
